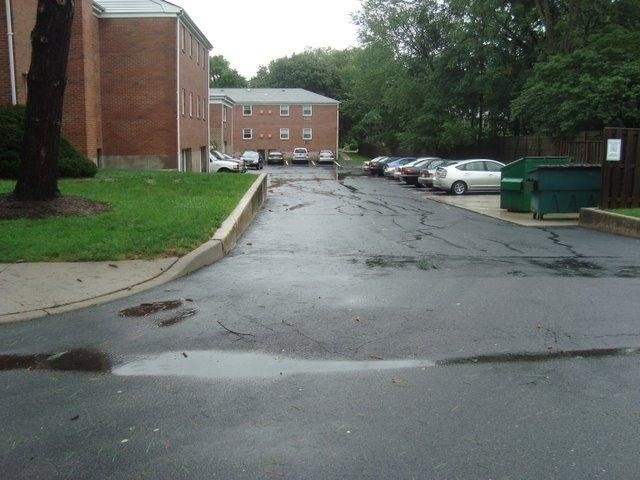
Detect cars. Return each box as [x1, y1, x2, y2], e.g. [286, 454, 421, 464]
[361, 155, 507, 194]
[239, 151, 263, 170]
[209, 149, 247, 173]
[267, 152, 283, 164]
[317, 149, 335, 162]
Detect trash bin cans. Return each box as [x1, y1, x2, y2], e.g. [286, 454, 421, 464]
[525, 164, 602, 221]
[499, 155, 571, 213]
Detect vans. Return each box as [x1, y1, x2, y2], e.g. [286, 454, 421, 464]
[292, 148, 309, 164]
